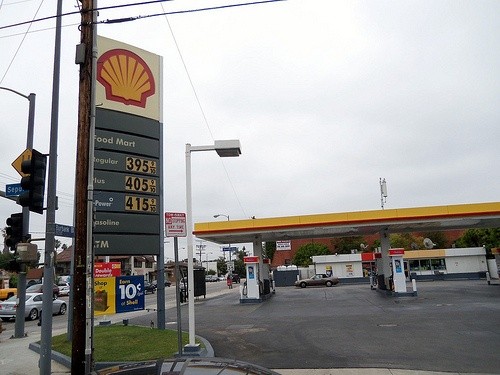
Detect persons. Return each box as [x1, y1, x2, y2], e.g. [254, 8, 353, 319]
[227, 274, 232, 289]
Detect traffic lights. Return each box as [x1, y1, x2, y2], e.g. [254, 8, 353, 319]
[6, 213, 24, 247]
[19, 148, 47, 214]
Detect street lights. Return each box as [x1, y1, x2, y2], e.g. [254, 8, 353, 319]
[213, 214, 231, 261]
[183, 139, 242, 352]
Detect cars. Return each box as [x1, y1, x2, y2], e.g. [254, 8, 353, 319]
[205, 273, 241, 284]
[152, 279, 172, 288]
[0, 275, 70, 301]
[0, 292, 68, 322]
[178, 277, 187, 292]
[144, 280, 157, 295]
[294, 273, 340, 288]
